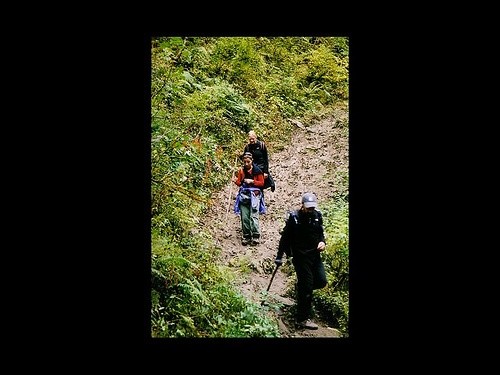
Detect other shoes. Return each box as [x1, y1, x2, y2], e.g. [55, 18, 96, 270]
[301, 319, 318, 330]
[241, 232, 252, 246]
[252, 233, 260, 244]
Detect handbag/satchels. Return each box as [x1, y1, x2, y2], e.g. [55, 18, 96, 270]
[238, 191, 251, 204]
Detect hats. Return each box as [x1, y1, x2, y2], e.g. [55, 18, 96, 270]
[302, 193, 317, 208]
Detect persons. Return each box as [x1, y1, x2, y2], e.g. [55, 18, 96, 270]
[275, 193, 327, 330]
[234, 131, 275, 192]
[230, 152, 264, 246]
